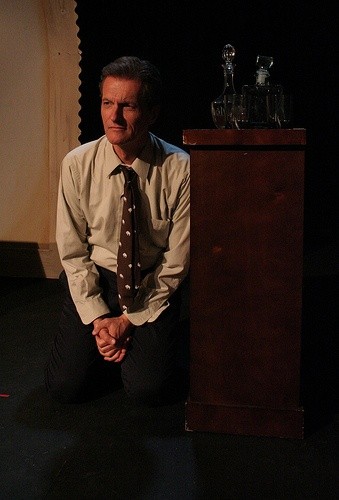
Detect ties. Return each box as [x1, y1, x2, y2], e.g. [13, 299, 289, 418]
[118, 167, 138, 314]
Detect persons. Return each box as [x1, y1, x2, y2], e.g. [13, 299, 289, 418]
[39, 56, 191, 421]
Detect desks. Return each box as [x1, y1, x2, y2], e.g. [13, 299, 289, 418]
[181, 124, 303, 444]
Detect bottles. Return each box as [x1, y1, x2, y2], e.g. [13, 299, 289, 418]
[211, 44, 249, 130]
[241, 54, 288, 130]
